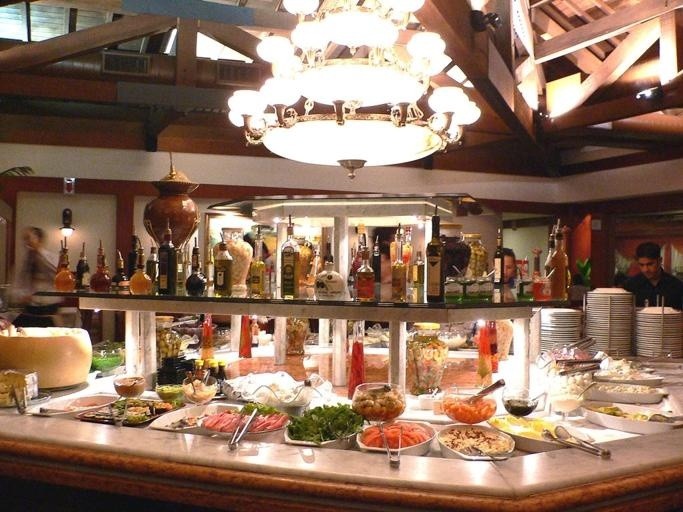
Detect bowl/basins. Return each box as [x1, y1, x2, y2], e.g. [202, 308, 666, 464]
[502, 396, 538, 416]
[438, 329, 468, 350]
[113, 376, 145, 398]
[352, 382, 407, 422]
[154, 375, 218, 407]
[443, 384, 500, 423]
[89, 345, 124, 373]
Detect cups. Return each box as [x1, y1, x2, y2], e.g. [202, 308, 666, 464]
[442, 277, 493, 304]
[515, 275, 552, 302]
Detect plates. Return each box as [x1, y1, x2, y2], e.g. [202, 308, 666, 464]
[583, 285, 634, 357]
[539, 307, 581, 352]
[634, 305, 682, 359]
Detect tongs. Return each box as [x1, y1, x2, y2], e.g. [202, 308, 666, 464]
[553, 358, 600, 376]
[466, 379, 506, 404]
[227, 405, 259, 450]
[376, 422, 402, 467]
[566, 335, 595, 352]
[108, 401, 129, 427]
[542, 423, 611, 460]
[8, 384, 29, 415]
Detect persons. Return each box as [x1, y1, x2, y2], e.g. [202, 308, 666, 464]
[364, 227, 411, 329]
[624, 240, 681, 310]
[244, 231, 272, 269]
[10, 224, 60, 328]
[470, 247, 517, 338]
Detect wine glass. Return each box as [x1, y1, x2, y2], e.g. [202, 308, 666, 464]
[549, 395, 583, 426]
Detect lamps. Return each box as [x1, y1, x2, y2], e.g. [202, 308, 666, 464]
[634, 84, 665, 102]
[226, 0, 483, 180]
[469, 8, 501, 33]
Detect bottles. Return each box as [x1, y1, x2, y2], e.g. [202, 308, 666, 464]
[154, 314, 181, 367]
[193, 357, 227, 394]
[520, 258, 529, 278]
[406, 323, 448, 397]
[51, 202, 446, 309]
[545, 217, 571, 302]
[442, 233, 488, 278]
[491, 236, 505, 284]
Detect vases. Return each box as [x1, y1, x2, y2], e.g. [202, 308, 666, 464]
[208, 225, 257, 291]
